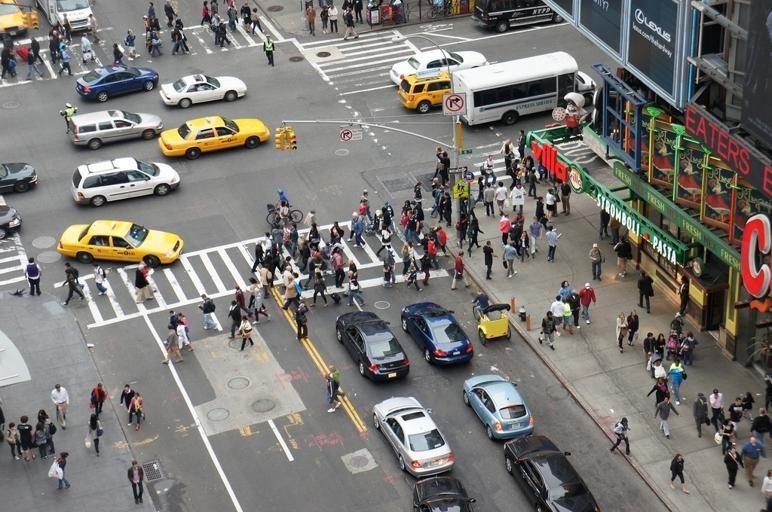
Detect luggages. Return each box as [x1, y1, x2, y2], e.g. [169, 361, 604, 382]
[330, 293, 340, 303]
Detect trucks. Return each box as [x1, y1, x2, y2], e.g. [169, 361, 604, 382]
[36, 0, 95, 34]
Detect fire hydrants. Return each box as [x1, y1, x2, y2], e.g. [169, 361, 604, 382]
[518, 306, 529, 321]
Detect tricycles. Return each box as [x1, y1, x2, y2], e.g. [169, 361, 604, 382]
[473, 302, 512, 347]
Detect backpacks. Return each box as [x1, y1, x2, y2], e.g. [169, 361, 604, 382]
[48, 422, 56, 434]
[98, 267, 105, 278]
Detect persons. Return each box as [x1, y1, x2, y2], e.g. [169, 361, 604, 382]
[59, 102, 78, 133]
[0, 0, 447, 80]
[563, 91, 589, 140]
[162, 130, 628, 415]
[1, 382, 146, 506]
[538, 237, 772, 511]
[26, 257, 157, 305]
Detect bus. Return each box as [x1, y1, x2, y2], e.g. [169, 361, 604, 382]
[451, 51, 597, 128]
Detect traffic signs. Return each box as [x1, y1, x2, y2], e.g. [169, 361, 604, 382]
[448, 167, 468, 175]
[462, 149, 473, 154]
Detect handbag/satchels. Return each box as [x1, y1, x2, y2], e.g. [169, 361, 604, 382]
[455, 272, 462, 279]
[706, 414, 724, 444]
[682, 371, 687, 379]
[267, 279, 272, 287]
[97, 429, 103, 436]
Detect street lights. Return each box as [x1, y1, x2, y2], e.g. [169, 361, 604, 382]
[393, 35, 460, 228]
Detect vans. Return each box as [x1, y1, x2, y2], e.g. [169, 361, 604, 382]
[68, 108, 162, 149]
[70, 157, 181, 207]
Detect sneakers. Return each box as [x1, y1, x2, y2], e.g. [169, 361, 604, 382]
[539, 320, 590, 350]
[328, 402, 341, 412]
[348, 239, 366, 248]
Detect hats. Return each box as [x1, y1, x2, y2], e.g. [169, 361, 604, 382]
[593, 244, 598, 247]
[585, 283, 590, 289]
[675, 311, 680, 319]
[277, 188, 283, 195]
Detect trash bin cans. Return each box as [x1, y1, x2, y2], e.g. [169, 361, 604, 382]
[366, 4, 381, 25]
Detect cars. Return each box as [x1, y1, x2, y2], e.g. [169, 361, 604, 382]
[390, 47, 488, 86]
[400, 301, 473, 369]
[0, 163, 38, 194]
[335, 311, 410, 381]
[462, 374, 534, 442]
[158, 73, 249, 108]
[57, 219, 186, 269]
[75, 63, 160, 104]
[0, 1, 28, 41]
[503, 434, 601, 512]
[412, 475, 475, 511]
[371, 396, 454, 477]
[0, 205, 23, 240]
[157, 115, 270, 160]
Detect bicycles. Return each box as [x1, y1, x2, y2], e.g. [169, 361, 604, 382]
[267, 205, 304, 226]
[426, 1, 454, 20]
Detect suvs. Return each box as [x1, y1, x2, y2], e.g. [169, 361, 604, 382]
[474, 0, 565, 32]
[397, 68, 454, 114]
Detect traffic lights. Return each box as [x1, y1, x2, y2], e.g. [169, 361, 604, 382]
[22, 14, 29, 29]
[31, 13, 40, 30]
[274, 126, 299, 150]
